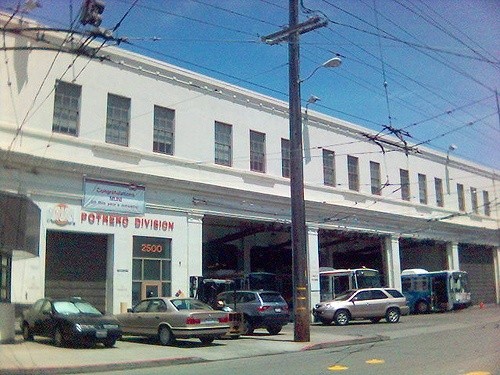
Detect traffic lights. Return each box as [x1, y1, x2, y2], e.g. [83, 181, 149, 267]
[79, 0, 106, 27]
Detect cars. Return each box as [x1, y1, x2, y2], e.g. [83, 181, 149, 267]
[116, 296, 232, 345]
[20, 297, 121, 347]
[313, 287, 410, 323]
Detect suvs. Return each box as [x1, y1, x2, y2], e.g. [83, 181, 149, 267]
[211, 289, 290, 334]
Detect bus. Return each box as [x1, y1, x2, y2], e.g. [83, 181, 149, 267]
[401, 268, 472, 315]
[206, 267, 282, 292]
[319, 268, 380, 301]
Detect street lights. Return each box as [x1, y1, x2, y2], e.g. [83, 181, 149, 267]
[285, 0, 344, 342]
